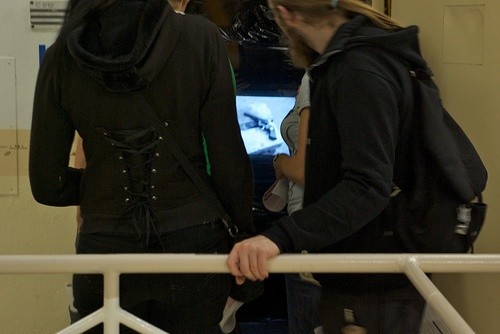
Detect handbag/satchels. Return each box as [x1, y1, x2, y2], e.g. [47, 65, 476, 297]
[226, 233, 266, 301]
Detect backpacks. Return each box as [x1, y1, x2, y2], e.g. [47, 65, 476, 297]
[379, 51, 488, 256]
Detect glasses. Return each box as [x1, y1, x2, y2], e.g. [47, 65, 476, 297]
[260, 5, 292, 20]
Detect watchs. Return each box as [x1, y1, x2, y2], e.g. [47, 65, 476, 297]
[272, 153, 288, 171]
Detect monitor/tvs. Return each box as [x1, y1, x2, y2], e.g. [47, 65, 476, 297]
[234, 90, 298, 159]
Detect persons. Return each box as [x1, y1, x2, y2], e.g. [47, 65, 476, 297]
[226, 0, 426, 334]
[28, 0, 255, 334]
